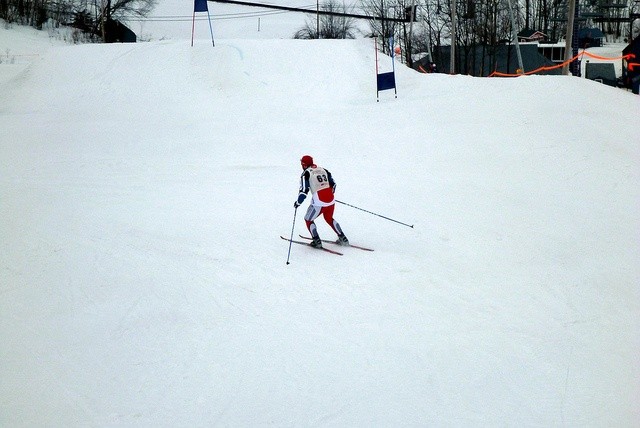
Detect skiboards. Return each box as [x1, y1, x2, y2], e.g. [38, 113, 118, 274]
[281, 233, 375, 255]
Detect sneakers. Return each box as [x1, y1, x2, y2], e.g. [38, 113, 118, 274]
[336, 236, 348, 244]
[311, 238, 321, 247]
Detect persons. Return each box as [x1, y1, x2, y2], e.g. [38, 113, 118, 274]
[294, 156, 348, 247]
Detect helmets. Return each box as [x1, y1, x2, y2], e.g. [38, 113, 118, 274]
[301, 156, 313, 165]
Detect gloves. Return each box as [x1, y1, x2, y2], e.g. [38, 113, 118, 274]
[294, 201, 300, 209]
